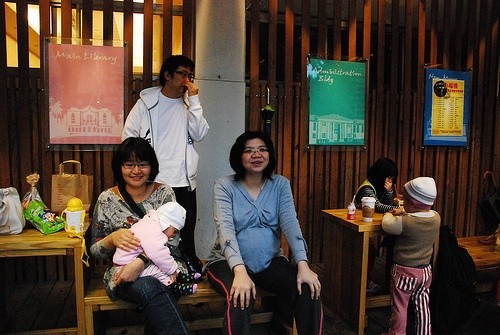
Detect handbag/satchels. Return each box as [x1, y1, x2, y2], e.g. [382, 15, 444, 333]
[0, 187, 26, 234]
[50, 159, 93, 212]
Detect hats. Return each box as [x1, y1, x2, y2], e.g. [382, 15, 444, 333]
[404, 177, 437, 205]
[155, 201, 186, 230]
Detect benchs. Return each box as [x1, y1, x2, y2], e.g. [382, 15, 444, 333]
[84, 280, 298, 335]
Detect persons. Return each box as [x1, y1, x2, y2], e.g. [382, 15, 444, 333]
[90, 137, 195, 335]
[113, 201, 201, 295]
[203, 130, 324, 335]
[351, 157, 404, 293]
[382, 176, 441, 335]
[122, 55, 209, 257]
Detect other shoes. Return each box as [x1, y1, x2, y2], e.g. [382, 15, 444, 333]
[366, 283, 381, 295]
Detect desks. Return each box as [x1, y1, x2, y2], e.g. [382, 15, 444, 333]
[0, 215, 92, 335]
[322, 209, 392, 335]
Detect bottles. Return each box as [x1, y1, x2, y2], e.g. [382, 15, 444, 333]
[60, 197, 85, 237]
[347, 202, 356, 220]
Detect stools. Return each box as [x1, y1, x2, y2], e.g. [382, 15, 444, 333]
[457, 235, 500, 306]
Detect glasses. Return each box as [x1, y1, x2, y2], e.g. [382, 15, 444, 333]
[172, 70, 194, 81]
[245, 146, 270, 156]
[122, 160, 152, 169]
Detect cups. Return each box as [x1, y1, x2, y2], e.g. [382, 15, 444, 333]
[361, 197, 376, 222]
